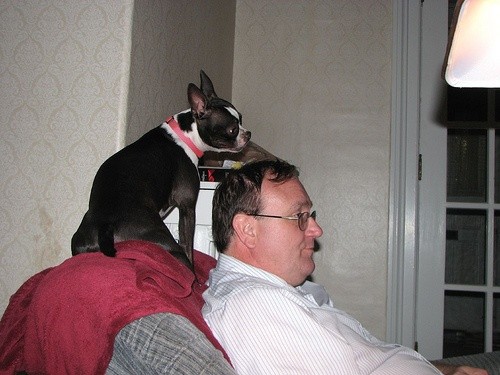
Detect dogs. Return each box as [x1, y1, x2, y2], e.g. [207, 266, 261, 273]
[71, 69, 252, 287]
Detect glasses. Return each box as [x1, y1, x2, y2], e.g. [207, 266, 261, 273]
[246, 211, 316, 231]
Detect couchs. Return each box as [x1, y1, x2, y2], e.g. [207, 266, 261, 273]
[0, 239, 498, 375]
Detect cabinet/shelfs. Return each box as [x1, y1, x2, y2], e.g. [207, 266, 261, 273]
[162, 181, 221, 260]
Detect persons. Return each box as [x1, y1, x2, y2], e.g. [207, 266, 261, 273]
[201, 159, 489, 375]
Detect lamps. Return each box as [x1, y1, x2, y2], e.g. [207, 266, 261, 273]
[444, 0, 500, 90]
